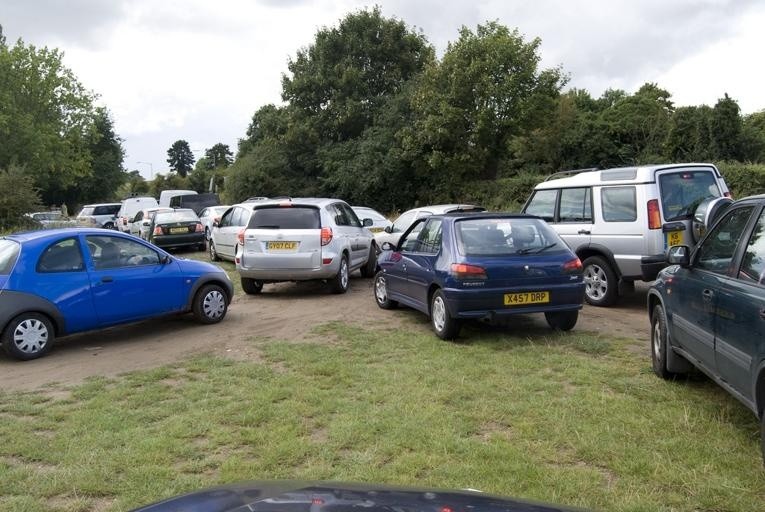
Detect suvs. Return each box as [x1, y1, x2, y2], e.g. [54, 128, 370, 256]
[520, 161, 732, 310]
[235, 193, 380, 295]
[77, 202, 122, 230]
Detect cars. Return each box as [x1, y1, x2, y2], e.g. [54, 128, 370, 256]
[371, 204, 485, 252]
[196, 206, 237, 239]
[642, 196, 765, 460]
[129, 207, 176, 241]
[0, 224, 232, 360]
[144, 209, 209, 252]
[374, 213, 589, 338]
[127, 483, 595, 511]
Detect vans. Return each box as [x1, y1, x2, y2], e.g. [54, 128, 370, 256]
[110, 197, 160, 232]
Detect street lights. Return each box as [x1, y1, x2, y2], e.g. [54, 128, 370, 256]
[135, 161, 153, 178]
